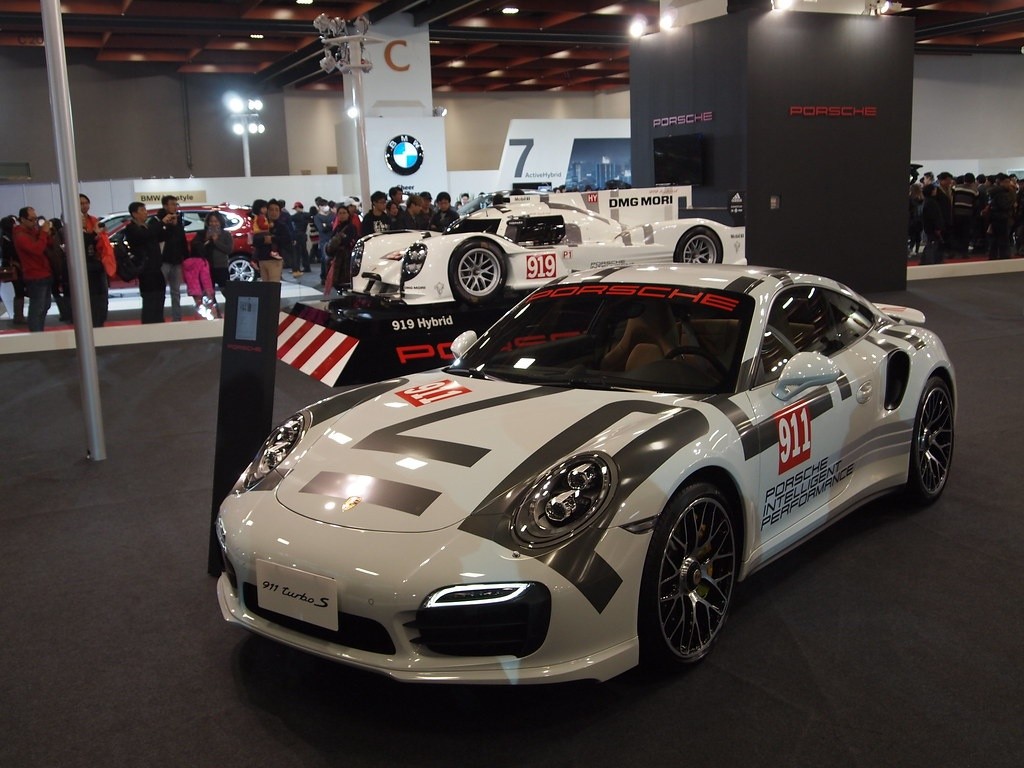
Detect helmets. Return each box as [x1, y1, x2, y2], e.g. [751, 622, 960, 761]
[344, 195, 362, 213]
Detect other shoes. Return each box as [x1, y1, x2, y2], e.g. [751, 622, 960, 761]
[301, 268, 311, 272]
[293, 271, 303, 277]
[269, 251, 283, 259]
[250, 261, 259, 271]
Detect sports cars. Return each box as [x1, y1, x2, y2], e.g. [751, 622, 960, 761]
[213, 262, 960, 689]
[334, 187, 748, 320]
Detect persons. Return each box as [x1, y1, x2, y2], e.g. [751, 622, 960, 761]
[910, 169, 1024, 264]
[0, 180, 631, 330]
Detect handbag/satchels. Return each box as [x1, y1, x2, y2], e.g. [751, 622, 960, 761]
[112, 239, 142, 281]
[192, 240, 211, 258]
[154, 216, 166, 255]
[94, 224, 117, 277]
[0, 260, 20, 282]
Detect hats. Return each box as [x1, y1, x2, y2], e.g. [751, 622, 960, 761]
[292, 202, 302, 209]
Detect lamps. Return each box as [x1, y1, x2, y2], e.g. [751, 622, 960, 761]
[433, 106, 448, 117]
[861, 0, 903, 17]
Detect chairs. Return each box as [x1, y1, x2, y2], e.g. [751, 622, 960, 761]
[601, 305, 710, 373]
[722, 302, 799, 368]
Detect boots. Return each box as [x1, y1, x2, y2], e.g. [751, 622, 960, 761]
[14, 297, 27, 323]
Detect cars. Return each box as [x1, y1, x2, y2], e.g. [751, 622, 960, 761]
[55, 202, 260, 291]
